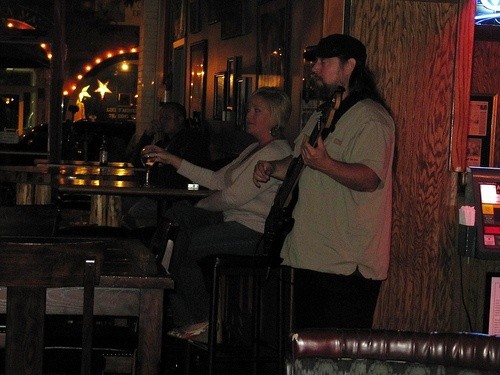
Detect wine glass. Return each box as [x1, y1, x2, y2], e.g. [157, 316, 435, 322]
[138, 146, 155, 190]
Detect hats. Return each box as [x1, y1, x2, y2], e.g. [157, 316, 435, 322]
[302, 33, 366, 61]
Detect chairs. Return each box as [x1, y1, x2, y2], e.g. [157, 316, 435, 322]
[0, 166, 64, 239]
[200, 254, 294, 375]
[0, 240, 106, 375]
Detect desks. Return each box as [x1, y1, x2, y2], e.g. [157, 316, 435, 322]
[16, 161, 221, 233]
[0, 239, 175, 375]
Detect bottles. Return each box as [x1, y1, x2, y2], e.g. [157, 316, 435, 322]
[99, 134, 109, 166]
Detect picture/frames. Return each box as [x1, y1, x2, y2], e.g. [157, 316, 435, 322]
[214, 56, 255, 126]
[468, 137, 490, 166]
[482, 272, 500, 336]
[469, 96, 493, 137]
[207, 0, 248, 40]
[189, 40, 209, 117]
[256, 0, 292, 96]
[189, 0, 200, 33]
[116, 91, 132, 108]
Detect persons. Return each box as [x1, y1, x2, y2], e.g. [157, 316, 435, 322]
[253, 34, 397, 329]
[133, 85, 291, 338]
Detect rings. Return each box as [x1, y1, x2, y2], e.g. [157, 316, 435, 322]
[142, 148, 145, 151]
[155, 153, 158, 157]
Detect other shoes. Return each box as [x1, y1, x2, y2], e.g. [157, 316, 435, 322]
[166, 321, 221, 339]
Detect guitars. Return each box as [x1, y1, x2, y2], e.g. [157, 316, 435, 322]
[260, 86, 343, 269]
